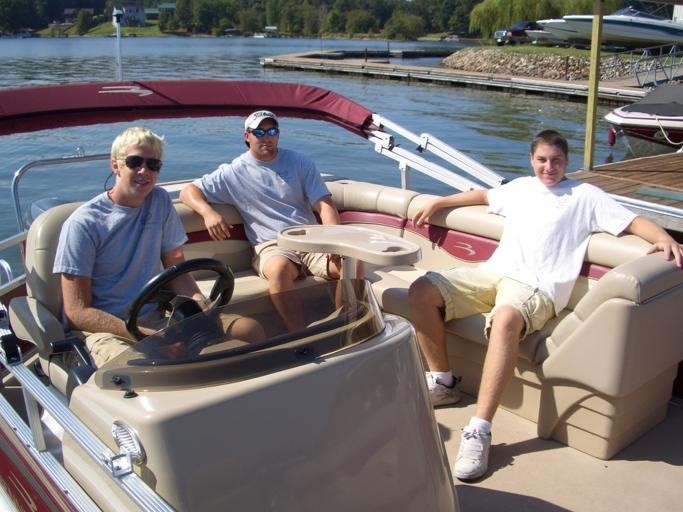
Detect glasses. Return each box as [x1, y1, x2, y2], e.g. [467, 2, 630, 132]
[116, 156, 162, 171]
[248, 127, 278, 137]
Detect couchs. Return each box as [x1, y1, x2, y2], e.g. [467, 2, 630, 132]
[7, 175, 683, 459]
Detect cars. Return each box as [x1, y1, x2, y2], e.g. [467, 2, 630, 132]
[494, 22, 538, 47]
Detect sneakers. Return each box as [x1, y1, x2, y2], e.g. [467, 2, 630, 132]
[425, 372, 462, 406]
[453, 425, 492, 480]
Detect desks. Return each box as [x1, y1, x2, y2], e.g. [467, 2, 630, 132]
[278, 225, 423, 281]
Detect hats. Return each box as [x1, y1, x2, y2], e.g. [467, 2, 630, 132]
[245, 111, 278, 133]
[164, 295, 217, 350]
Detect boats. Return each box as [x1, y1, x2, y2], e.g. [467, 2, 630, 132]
[562, 7, 682, 50]
[525, 30, 558, 46]
[598, 80, 682, 150]
[0, 83, 682, 512]
[536, 20, 581, 41]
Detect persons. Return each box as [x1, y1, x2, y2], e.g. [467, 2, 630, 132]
[405, 128, 682, 480]
[50, 127, 268, 367]
[174, 111, 366, 353]
